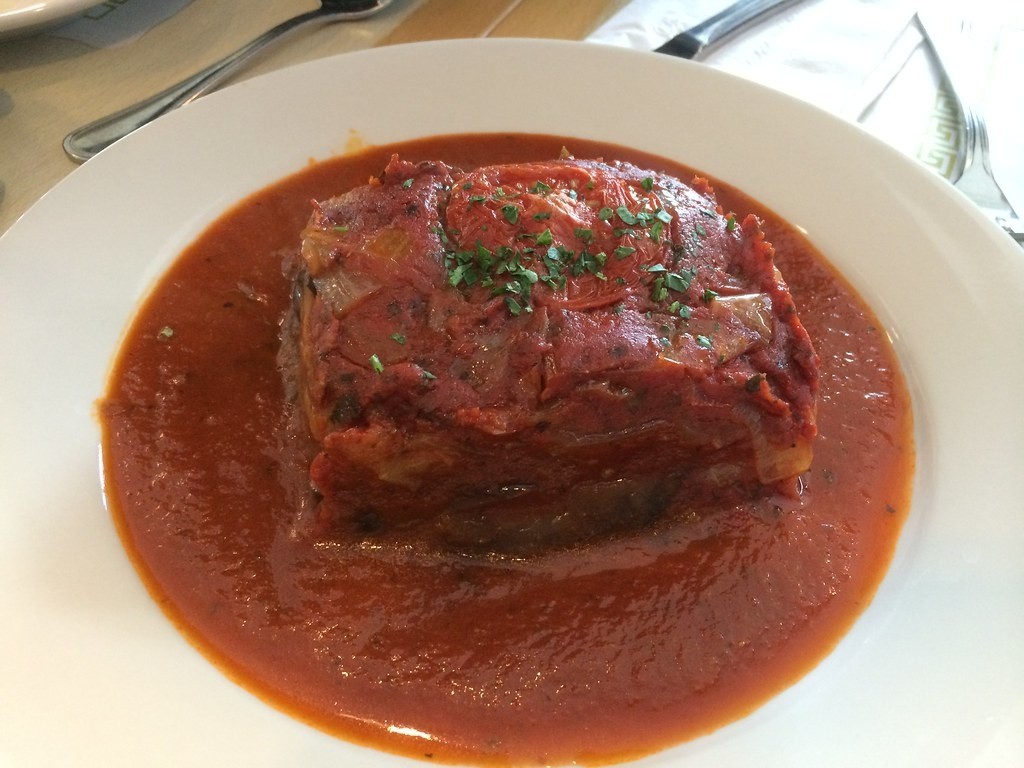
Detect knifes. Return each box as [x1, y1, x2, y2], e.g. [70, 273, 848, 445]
[649, 0, 813, 62]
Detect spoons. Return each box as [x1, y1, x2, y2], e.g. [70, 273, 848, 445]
[61, 0, 396, 164]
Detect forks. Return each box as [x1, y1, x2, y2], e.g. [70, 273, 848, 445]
[915, 8, 1024, 241]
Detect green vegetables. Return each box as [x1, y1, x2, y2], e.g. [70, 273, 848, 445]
[330, 177, 737, 378]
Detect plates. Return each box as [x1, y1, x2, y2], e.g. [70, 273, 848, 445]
[1, 38, 1024, 767]
